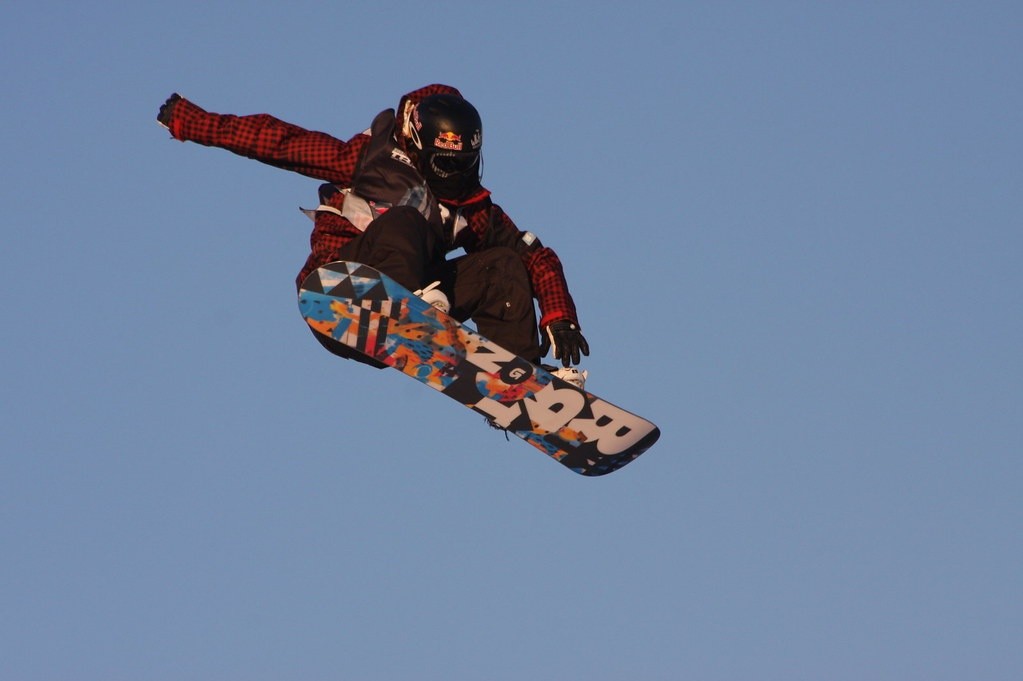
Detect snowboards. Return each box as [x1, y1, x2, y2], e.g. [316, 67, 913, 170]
[297, 258, 663, 477]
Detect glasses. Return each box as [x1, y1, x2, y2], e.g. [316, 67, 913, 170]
[430, 151, 479, 178]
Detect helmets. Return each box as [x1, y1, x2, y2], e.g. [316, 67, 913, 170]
[407, 93, 482, 200]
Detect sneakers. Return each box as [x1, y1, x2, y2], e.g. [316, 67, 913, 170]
[413, 280, 450, 316]
[547, 366, 588, 390]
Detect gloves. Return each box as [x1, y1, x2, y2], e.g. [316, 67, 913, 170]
[539, 318, 590, 368]
[156, 92, 181, 128]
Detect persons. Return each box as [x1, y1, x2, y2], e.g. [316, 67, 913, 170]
[157, 84, 590, 390]
